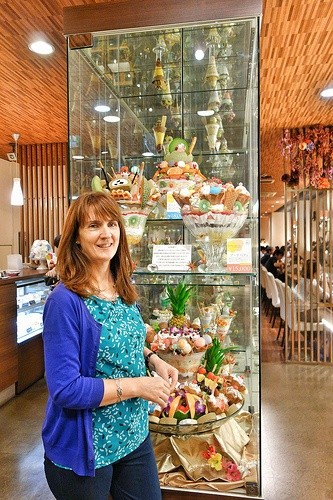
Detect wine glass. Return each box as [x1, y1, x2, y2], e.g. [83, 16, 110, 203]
[180, 208, 250, 275]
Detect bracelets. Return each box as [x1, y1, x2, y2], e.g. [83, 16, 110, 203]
[114, 378, 122, 403]
[145, 352, 156, 370]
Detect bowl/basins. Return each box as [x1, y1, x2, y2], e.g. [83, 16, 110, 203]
[148, 393, 246, 437]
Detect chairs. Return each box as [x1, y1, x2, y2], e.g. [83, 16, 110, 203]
[260, 263, 321, 358]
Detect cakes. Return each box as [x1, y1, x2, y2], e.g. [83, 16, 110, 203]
[29, 240, 53, 259]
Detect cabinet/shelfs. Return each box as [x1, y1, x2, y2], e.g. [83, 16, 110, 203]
[66, 18, 259, 500]
[16, 267, 51, 392]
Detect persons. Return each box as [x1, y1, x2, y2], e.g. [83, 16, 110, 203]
[260, 245, 333, 358]
[41, 191, 179, 500]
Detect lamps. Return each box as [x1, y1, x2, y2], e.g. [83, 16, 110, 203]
[10, 133, 25, 205]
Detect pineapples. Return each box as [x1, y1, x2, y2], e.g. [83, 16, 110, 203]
[164, 278, 197, 328]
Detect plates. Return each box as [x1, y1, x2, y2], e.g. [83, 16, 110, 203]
[156, 178, 196, 194]
[5, 270, 21, 276]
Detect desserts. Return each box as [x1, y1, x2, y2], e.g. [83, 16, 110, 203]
[199, 303, 236, 343]
[140, 319, 245, 434]
[89, 137, 250, 245]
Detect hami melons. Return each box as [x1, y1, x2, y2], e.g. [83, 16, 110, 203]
[168, 395, 182, 419]
[184, 393, 196, 419]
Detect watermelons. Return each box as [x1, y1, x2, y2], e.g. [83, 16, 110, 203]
[173, 401, 204, 420]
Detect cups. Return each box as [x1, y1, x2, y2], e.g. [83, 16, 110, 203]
[114, 206, 152, 254]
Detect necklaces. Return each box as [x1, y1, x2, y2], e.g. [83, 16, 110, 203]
[100, 286, 117, 305]
